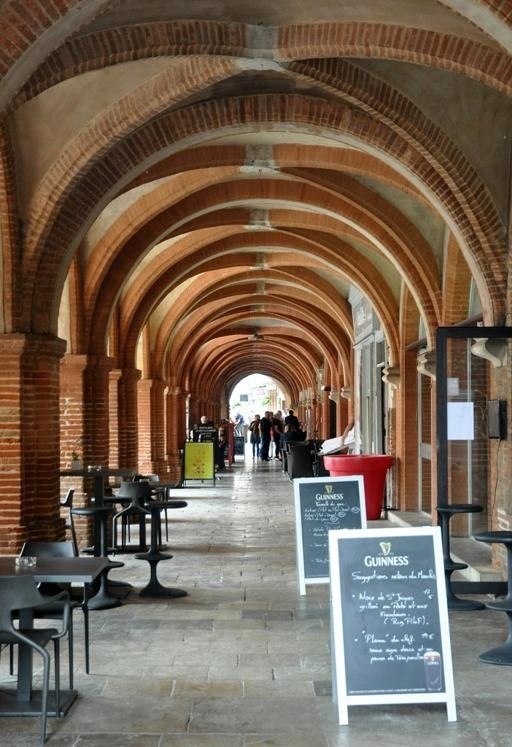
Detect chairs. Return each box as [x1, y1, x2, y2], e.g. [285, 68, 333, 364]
[60, 489, 79, 556]
[281, 439, 329, 480]
[111, 474, 169, 554]
[0, 541, 90, 744]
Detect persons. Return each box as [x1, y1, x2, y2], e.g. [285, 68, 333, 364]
[215, 418, 229, 473]
[269, 412, 274, 459]
[248, 414, 260, 457]
[257, 411, 271, 462]
[197, 414, 215, 428]
[340, 416, 354, 454]
[271, 409, 284, 461]
[233, 409, 245, 436]
[280, 423, 290, 447]
[284, 409, 300, 426]
[285, 423, 305, 442]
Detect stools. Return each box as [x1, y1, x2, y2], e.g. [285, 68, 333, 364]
[70, 500, 187, 611]
[437, 502, 482, 612]
[474, 528, 512, 666]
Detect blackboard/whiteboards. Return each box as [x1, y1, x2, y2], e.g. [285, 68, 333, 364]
[294, 475, 367, 584]
[329, 526, 453, 706]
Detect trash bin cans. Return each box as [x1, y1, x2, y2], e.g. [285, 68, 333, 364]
[285, 442, 314, 478]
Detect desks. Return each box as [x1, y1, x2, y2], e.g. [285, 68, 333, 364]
[0, 557, 108, 718]
[59, 468, 135, 557]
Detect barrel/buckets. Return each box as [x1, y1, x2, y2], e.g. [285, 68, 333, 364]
[324, 454, 396, 519]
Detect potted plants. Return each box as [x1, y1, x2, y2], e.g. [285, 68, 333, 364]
[71, 450, 82, 471]
